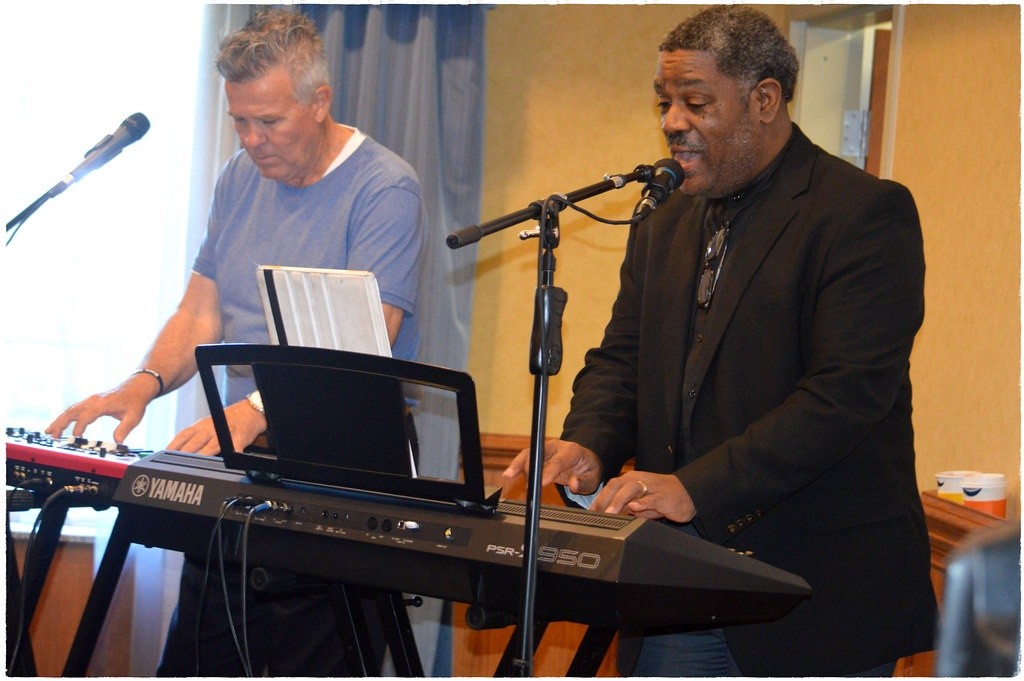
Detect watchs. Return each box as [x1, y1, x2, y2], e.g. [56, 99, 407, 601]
[245, 390, 265, 414]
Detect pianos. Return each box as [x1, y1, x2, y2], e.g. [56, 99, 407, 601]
[5, 419, 815, 675]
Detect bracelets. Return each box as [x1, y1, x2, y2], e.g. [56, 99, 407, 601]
[131, 369, 163, 398]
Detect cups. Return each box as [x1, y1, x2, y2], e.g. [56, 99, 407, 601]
[934, 471, 975, 505]
[960, 473, 1008, 520]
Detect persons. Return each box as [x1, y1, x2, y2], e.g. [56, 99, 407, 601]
[44, 9, 429, 677]
[502, 4, 943, 678]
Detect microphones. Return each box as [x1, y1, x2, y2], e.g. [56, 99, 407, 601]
[636, 157, 685, 221]
[47, 111, 151, 198]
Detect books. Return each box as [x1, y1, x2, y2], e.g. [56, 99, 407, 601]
[256, 266, 418, 479]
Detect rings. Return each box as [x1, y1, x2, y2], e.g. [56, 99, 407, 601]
[638, 480, 647, 498]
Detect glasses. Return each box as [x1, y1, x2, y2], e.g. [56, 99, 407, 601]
[696, 223, 730, 309]
